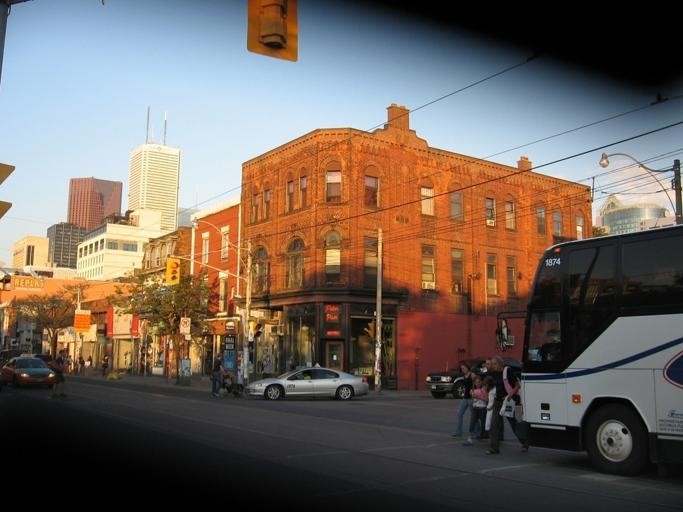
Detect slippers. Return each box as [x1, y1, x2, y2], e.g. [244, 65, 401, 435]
[521, 442, 529, 452]
[485, 447, 500, 455]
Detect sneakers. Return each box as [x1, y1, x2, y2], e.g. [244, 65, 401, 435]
[449, 431, 479, 446]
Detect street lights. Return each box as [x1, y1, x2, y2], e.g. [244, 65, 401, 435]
[598, 151, 682, 224]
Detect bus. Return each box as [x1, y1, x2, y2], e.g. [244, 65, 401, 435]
[516, 222, 683, 476]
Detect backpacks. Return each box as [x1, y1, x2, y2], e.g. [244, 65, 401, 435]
[503, 365, 522, 395]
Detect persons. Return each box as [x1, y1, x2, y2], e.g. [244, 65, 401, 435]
[304, 372, 311, 379]
[236, 359, 244, 394]
[102, 354, 109, 377]
[452, 356, 530, 455]
[212, 353, 226, 398]
[50, 350, 92, 400]
[536, 329, 560, 360]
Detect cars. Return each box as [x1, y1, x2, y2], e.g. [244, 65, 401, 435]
[244, 367, 370, 401]
[425, 356, 521, 399]
[1, 356, 57, 388]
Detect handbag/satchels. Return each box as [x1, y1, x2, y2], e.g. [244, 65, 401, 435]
[499, 396, 516, 418]
